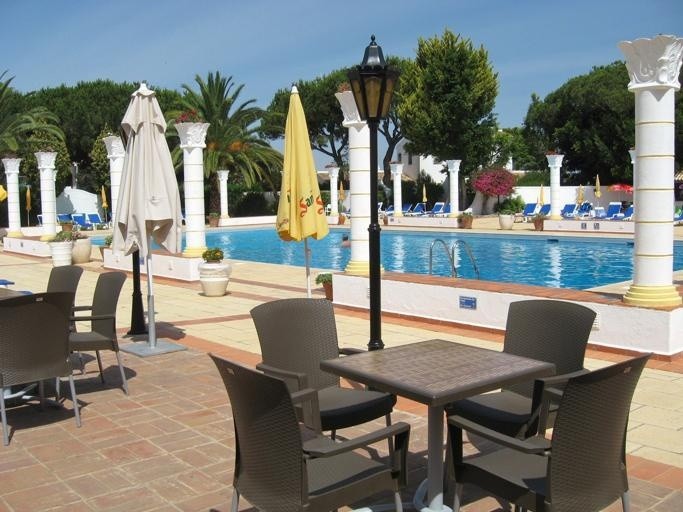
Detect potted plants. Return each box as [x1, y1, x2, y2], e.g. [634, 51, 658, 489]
[532, 214, 545, 231]
[98, 235, 112, 262]
[72, 233, 91, 263]
[315, 274, 332, 300]
[462, 212, 473, 229]
[499, 210, 514, 230]
[48, 232, 74, 267]
[197, 249, 230, 296]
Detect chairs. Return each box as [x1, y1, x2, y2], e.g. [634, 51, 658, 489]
[342, 202, 450, 221]
[208, 352, 410, 512]
[37, 211, 108, 231]
[47, 265, 85, 374]
[54, 271, 129, 405]
[515, 203, 683, 225]
[445, 299, 596, 436]
[250, 298, 397, 457]
[0, 291, 81, 447]
[449, 353, 653, 511]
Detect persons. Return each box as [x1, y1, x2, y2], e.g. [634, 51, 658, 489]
[338, 212, 345, 225]
[627, 202, 633, 208]
[383, 214, 388, 224]
[675, 205, 683, 216]
[621, 201, 627, 211]
[341, 234, 351, 247]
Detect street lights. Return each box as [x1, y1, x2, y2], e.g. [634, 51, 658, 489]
[347, 35, 400, 350]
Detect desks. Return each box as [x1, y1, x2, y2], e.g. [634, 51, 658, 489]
[0, 288, 27, 302]
[320, 339, 555, 512]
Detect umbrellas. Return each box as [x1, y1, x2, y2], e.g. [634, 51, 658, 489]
[26, 184, 32, 226]
[608, 183, 633, 202]
[102, 185, 109, 223]
[111, 81, 189, 347]
[422, 183, 428, 215]
[538, 184, 544, 207]
[595, 174, 602, 207]
[339, 180, 345, 211]
[577, 185, 584, 208]
[276, 85, 329, 298]
